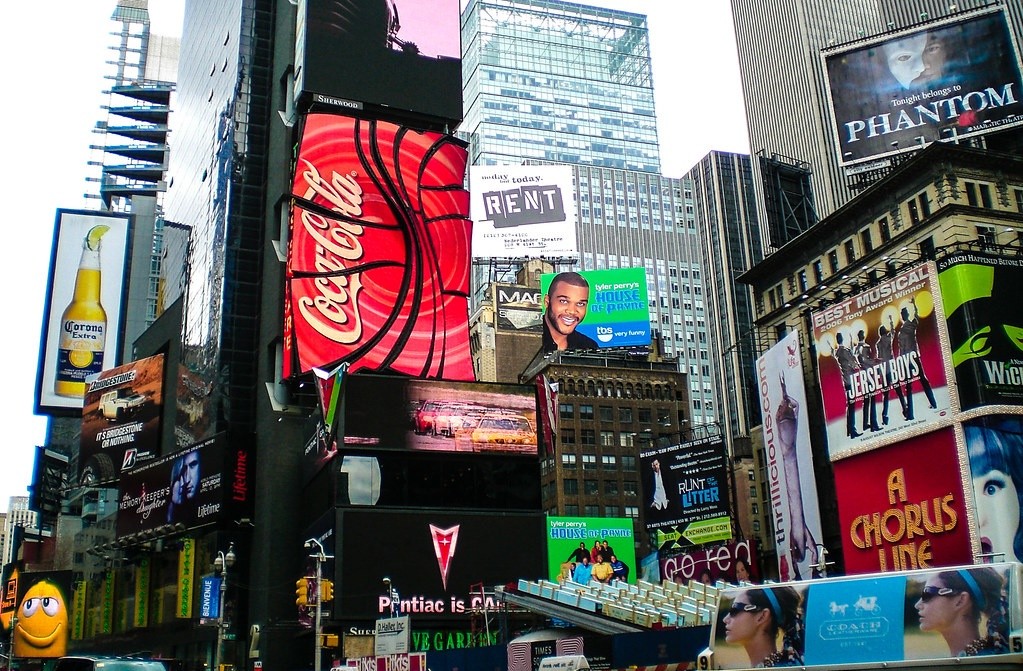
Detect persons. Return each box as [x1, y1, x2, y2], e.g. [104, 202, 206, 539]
[850, 329, 885, 432]
[561, 536, 630, 590]
[875, 312, 911, 425]
[896, 293, 937, 422]
[181, 450, 205, 526]
[881, 27, 980, 143]
[963, 420, 1022, 566]
[541, 270, 601, 353]
[912, 569, 1015, 659]
[646, 456, 681, 522]
[721, 586, 806, 669]
[166, 457, 191, 527]
[825, 332, 863, 440]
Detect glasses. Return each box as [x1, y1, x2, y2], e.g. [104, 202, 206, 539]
[920, 586, 963, 603]
[729, 603, 764, 618]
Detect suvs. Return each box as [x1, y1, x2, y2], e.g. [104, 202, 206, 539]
[96, 387, 155, 425]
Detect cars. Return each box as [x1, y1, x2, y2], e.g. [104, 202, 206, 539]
[410, 397, 538, 455]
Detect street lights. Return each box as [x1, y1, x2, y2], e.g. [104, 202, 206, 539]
[210, 540, 235, 671]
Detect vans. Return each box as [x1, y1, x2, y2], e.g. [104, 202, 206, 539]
[53, 654, 166, 671]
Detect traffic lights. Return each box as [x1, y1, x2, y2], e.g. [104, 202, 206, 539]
[320, 579, 334, 602]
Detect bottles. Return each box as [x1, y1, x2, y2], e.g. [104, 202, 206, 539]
[53, 236, 108, 399]
